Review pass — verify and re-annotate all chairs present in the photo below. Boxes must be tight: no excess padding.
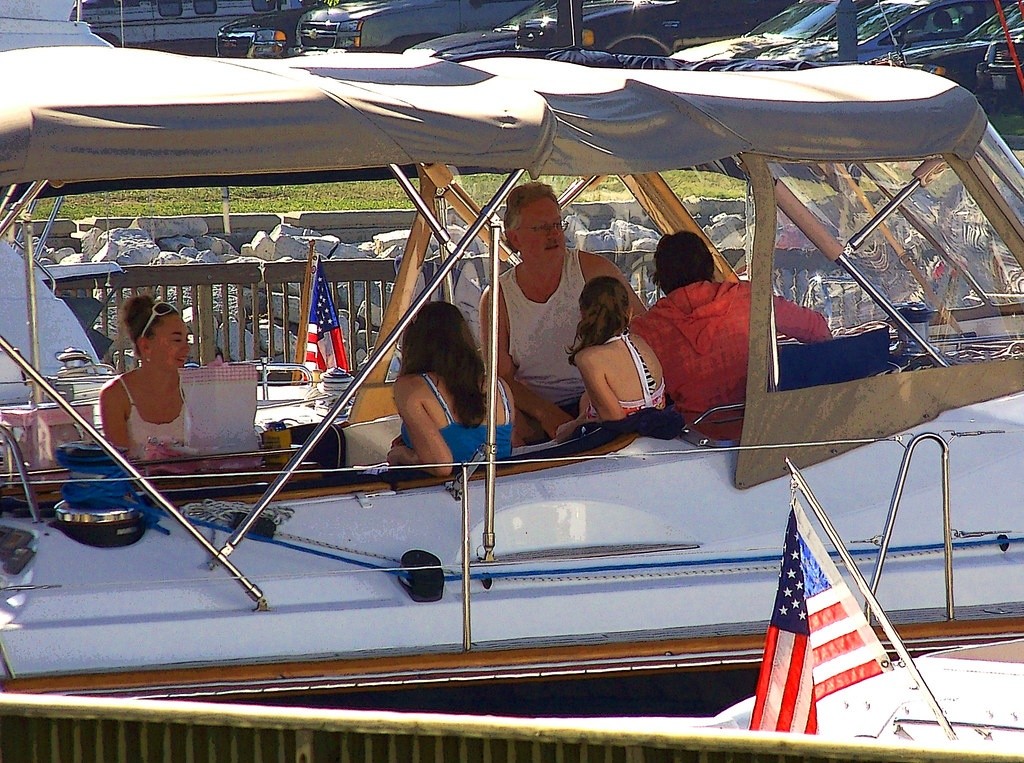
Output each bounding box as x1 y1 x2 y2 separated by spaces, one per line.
772 326 892 394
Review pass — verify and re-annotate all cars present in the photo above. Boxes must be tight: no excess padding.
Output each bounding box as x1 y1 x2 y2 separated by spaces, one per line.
664 0 1024 117
400 0 808 66
215 4 324 57
296 0 550 52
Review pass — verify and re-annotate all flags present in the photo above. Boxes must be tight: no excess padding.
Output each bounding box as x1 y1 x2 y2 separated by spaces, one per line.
302 252 351 380
749 497 892 734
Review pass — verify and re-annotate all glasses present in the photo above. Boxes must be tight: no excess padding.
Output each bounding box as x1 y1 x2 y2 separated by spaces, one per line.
514 220 569 233
141 302 180 338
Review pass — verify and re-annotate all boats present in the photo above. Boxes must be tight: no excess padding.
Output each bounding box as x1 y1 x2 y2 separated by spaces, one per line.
1 40 1024 753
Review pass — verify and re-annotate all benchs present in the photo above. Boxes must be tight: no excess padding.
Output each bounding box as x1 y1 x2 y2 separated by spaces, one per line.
1 406 684 515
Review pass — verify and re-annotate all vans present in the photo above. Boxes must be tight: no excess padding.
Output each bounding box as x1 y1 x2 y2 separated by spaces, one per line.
64 1 327 54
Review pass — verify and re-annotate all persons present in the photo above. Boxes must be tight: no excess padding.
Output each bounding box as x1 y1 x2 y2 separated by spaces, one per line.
632 232 834 441
387 302 516 479
557 275 667 440
100 296 190 450
480 182 645 447
